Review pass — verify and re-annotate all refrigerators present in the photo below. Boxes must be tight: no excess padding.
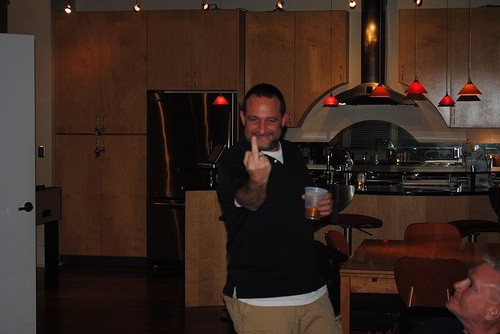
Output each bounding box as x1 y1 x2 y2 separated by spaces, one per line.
146 88 236 295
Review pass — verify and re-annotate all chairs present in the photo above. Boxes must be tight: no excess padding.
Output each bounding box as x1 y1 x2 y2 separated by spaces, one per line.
323 221 472 334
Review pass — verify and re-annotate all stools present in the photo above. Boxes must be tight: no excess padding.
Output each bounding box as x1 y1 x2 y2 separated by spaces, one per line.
329 213 383 247
449 219 500 242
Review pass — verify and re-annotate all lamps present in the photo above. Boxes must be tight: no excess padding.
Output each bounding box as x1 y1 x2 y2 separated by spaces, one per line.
213 92 229 105
369 0 392 98
202 2 217 9
438 0 456 106
63 3 72 14
275 1 284 12
133 2 141 11
320 0 340 107
402 5 428 95
457 0 483 96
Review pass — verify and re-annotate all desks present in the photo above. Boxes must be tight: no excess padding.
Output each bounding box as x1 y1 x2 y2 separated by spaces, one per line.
339 238 500 334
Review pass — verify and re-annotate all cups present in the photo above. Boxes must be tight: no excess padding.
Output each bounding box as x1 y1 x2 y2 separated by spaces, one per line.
357 174 365 191
304 187 329 220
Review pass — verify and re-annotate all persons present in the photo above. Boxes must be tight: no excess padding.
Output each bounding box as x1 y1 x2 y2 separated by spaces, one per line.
408 255 500 334
215 83 340 334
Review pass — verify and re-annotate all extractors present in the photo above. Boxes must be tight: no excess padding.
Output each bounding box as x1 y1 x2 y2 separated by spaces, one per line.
322 0 419 108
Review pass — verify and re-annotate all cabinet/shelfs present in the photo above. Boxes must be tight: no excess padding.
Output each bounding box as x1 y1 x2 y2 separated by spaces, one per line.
50 9 499 310
398 8 500 127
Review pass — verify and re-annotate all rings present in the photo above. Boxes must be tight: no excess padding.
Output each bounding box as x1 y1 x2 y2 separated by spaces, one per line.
259 155 264 157
